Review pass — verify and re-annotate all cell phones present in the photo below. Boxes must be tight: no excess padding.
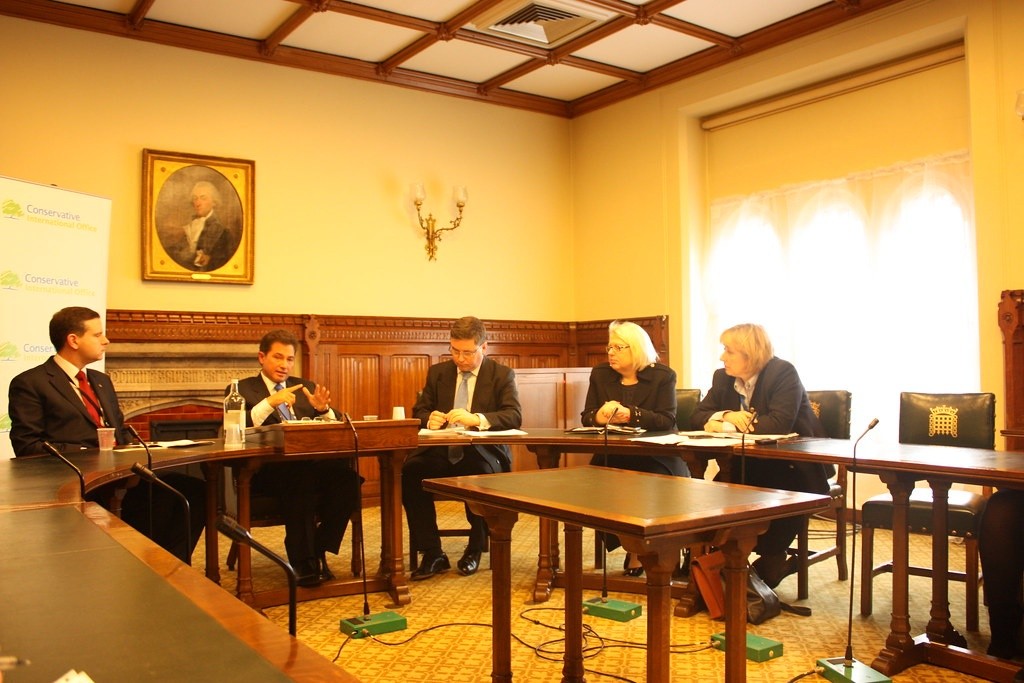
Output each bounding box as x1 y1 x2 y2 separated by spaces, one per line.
756 439 776 444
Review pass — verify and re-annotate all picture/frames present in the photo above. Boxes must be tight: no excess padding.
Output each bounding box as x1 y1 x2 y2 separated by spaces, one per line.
141 148 255 285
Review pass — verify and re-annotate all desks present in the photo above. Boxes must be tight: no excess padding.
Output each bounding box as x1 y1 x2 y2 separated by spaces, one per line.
420 465 831 683
0 418 1024 683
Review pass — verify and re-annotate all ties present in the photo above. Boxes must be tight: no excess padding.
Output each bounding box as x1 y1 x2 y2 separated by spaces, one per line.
275 383 294 422
76 370 101 426
448 371 473 464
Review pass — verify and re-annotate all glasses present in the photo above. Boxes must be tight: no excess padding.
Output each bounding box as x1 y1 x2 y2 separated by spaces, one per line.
605 344 630 354
448 344 485 359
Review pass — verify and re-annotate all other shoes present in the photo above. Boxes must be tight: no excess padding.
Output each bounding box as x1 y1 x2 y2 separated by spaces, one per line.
299 573 321 588
316 550 336 578
627 551 645 578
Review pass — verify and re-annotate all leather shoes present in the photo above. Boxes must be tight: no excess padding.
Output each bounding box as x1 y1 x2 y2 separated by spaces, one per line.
410 549 450 580
457 546 482 576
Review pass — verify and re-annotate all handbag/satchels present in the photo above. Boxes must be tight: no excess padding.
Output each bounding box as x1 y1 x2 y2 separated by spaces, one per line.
693 544 725 619
744 564 813 625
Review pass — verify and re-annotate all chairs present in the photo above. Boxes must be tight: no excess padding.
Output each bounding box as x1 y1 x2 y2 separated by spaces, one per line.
214 384 996 633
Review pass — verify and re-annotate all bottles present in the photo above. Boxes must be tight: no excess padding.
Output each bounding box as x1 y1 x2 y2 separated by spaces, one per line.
224 379 246 442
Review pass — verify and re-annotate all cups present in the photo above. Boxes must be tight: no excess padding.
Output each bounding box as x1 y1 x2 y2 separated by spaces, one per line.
363 415 378 421
393 406 405 420
223 424 242 447
97 428 116 450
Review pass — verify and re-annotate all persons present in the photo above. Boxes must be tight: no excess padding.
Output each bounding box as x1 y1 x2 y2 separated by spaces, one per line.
689 323 837 576
9 306 207 559
402 316 523 580
581 322 691 576
225 330 358 586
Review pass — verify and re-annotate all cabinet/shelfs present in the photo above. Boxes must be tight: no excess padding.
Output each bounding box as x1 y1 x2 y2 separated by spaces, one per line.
512 367 599 474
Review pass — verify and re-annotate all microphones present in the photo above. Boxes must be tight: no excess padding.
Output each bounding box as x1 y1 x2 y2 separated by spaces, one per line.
43 425 298 636
602 408 618 603
344 411 369 616
741 412 757 484
845 418 879 665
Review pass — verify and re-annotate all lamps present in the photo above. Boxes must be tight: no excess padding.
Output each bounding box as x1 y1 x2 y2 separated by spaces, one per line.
411 183 468 260
